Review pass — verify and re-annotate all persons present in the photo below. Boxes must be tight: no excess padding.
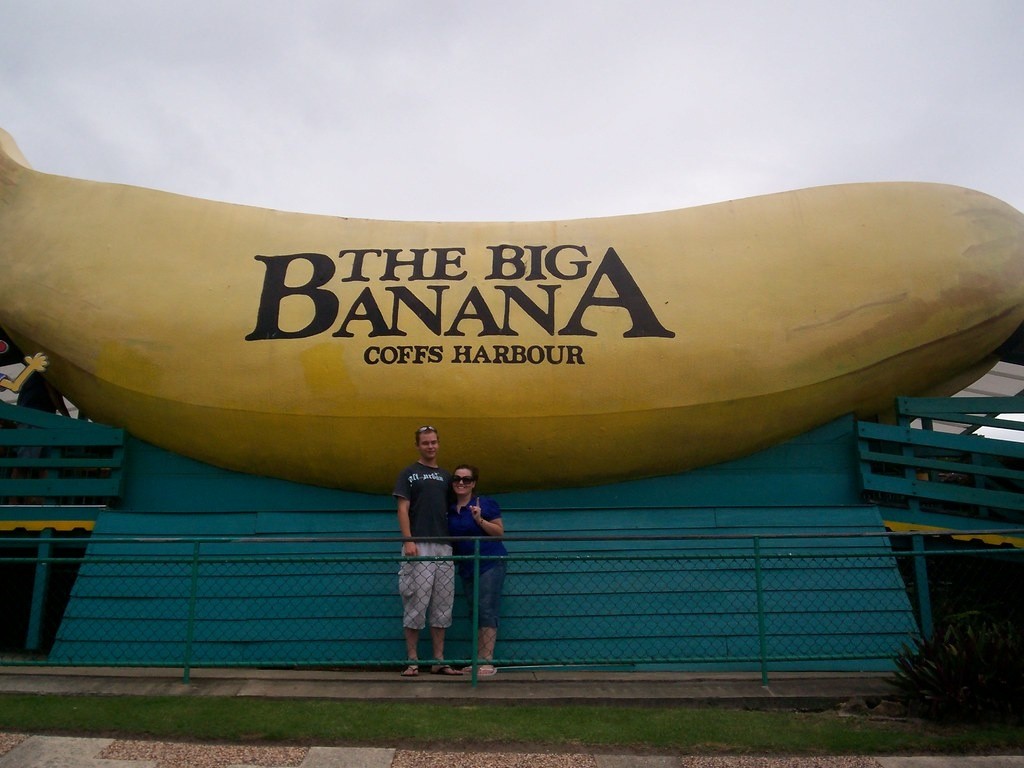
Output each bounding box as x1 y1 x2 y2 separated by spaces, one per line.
9 368 72 504
448 463 507 677
392 424 461 676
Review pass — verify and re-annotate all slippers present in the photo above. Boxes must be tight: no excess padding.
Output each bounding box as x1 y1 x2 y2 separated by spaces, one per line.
404 667 419 676
463 666 472 674
477 668 497 676
431 666 463 675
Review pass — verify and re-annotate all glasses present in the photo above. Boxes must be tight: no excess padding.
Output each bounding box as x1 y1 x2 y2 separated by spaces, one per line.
451 476 474 484
418 426 437 432
